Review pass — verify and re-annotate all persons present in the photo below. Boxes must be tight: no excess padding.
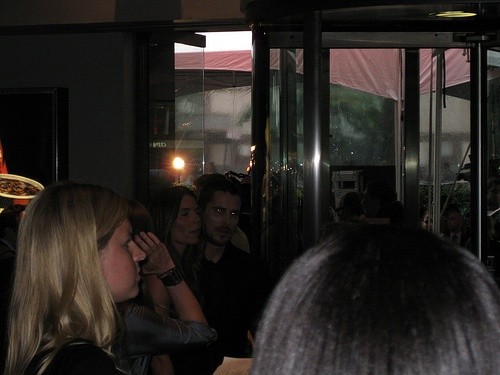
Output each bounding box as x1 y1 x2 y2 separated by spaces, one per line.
0 172 251 375
253 223 500 375
442 172 500 250
